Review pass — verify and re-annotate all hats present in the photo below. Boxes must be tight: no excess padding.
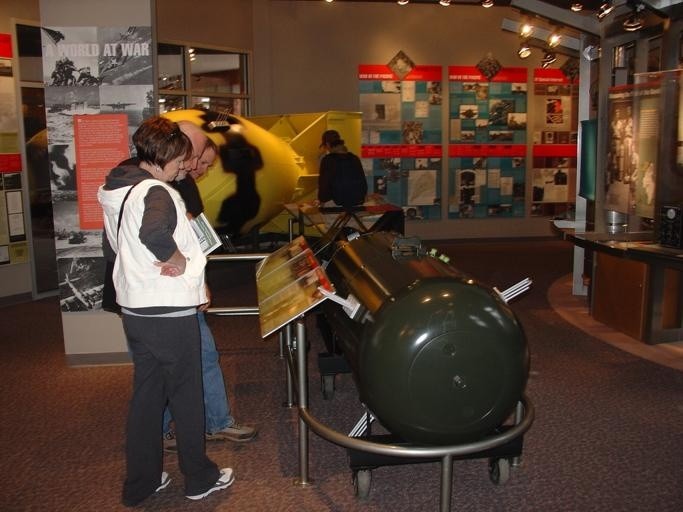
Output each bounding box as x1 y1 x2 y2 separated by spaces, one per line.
318 130 340 149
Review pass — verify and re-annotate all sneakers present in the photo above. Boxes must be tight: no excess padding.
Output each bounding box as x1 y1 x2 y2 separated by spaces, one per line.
121 472 172 507
185 467 236 501
162 430 178 454
206 420 257 443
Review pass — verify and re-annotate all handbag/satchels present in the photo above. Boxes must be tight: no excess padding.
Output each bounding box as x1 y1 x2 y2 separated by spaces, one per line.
101 248 121 315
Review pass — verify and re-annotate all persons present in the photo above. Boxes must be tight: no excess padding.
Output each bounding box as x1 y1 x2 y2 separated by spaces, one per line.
621 105 634 184
189 137 219 182
49 56 98 87
609 108 622 184
163 120 258 455
95 116 236 502
623 145 640 187
315 128 368 208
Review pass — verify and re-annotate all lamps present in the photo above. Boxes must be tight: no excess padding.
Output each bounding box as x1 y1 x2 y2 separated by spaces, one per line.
396 0 645 66
